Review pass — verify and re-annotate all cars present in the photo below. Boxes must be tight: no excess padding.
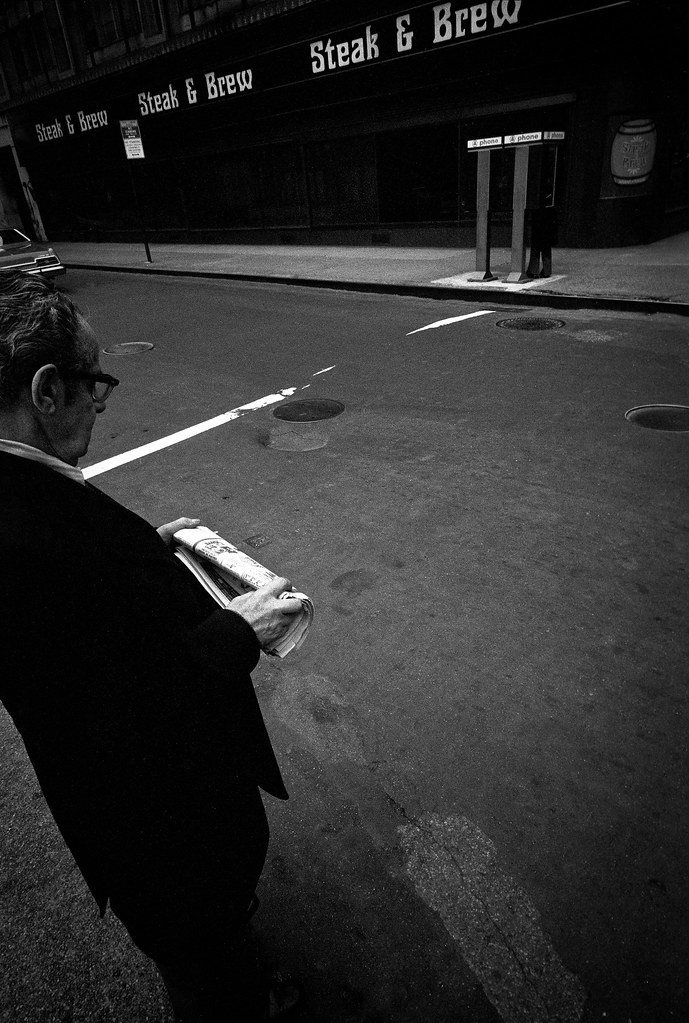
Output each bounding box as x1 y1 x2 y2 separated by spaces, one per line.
0 227 67 287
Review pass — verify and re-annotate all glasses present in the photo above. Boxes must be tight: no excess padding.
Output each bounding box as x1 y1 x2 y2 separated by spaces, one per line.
58 367 120 402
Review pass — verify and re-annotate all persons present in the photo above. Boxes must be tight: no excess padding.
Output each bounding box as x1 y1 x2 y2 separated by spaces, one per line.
0 266 311 1021
